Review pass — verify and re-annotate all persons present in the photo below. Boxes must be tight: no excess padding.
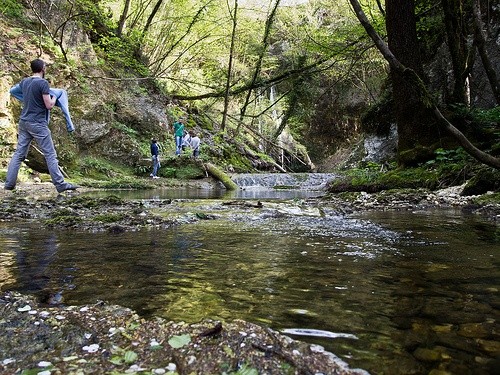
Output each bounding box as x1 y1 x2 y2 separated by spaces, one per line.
191 133 200 157
182 130 193 149
150 139 161 179
172 117 184 156
4 59 78 193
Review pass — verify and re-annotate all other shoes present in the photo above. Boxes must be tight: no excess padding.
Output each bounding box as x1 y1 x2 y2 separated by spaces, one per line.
150 173 160 178
4 187 14 191
56 183 81 193
